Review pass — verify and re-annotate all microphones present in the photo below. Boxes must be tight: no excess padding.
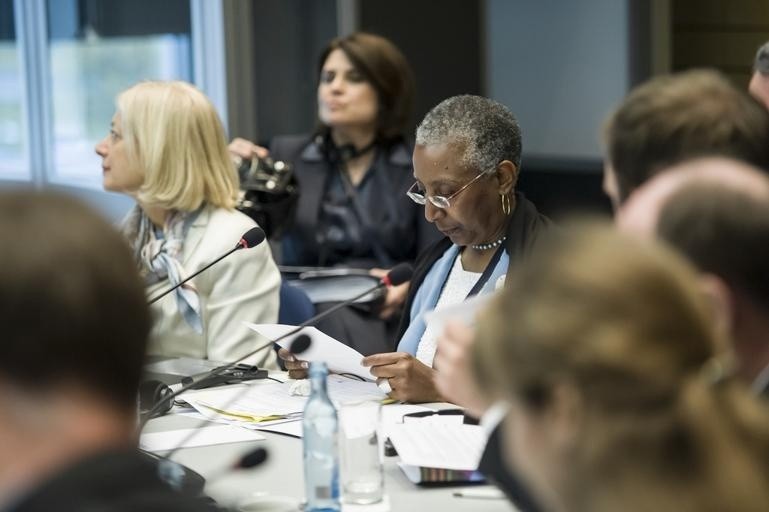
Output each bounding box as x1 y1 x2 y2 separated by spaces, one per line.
131 262 413 450
165 334 311 460
208 446 266 485
145 226 266 309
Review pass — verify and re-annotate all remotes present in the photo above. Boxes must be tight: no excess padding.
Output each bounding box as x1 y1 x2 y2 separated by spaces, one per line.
182 364 268 388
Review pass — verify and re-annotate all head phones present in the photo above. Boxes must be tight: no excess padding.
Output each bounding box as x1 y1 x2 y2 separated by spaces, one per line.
140 380 175 423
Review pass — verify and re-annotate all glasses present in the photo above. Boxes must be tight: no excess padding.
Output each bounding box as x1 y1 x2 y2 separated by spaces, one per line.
407 169 487 209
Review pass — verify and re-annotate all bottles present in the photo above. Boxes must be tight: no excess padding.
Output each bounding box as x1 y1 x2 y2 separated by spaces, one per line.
302 362 340 512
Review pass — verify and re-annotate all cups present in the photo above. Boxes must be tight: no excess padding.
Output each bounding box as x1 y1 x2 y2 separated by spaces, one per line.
341 403 384 505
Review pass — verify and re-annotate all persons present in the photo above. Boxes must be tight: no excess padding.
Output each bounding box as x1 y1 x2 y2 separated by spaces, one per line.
431 47 767 511
228 35 442 269
360 96 545 405
2 180 229 511
96 80 281 356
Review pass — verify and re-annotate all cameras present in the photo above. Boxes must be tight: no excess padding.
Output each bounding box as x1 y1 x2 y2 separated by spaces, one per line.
234 153 299 239
